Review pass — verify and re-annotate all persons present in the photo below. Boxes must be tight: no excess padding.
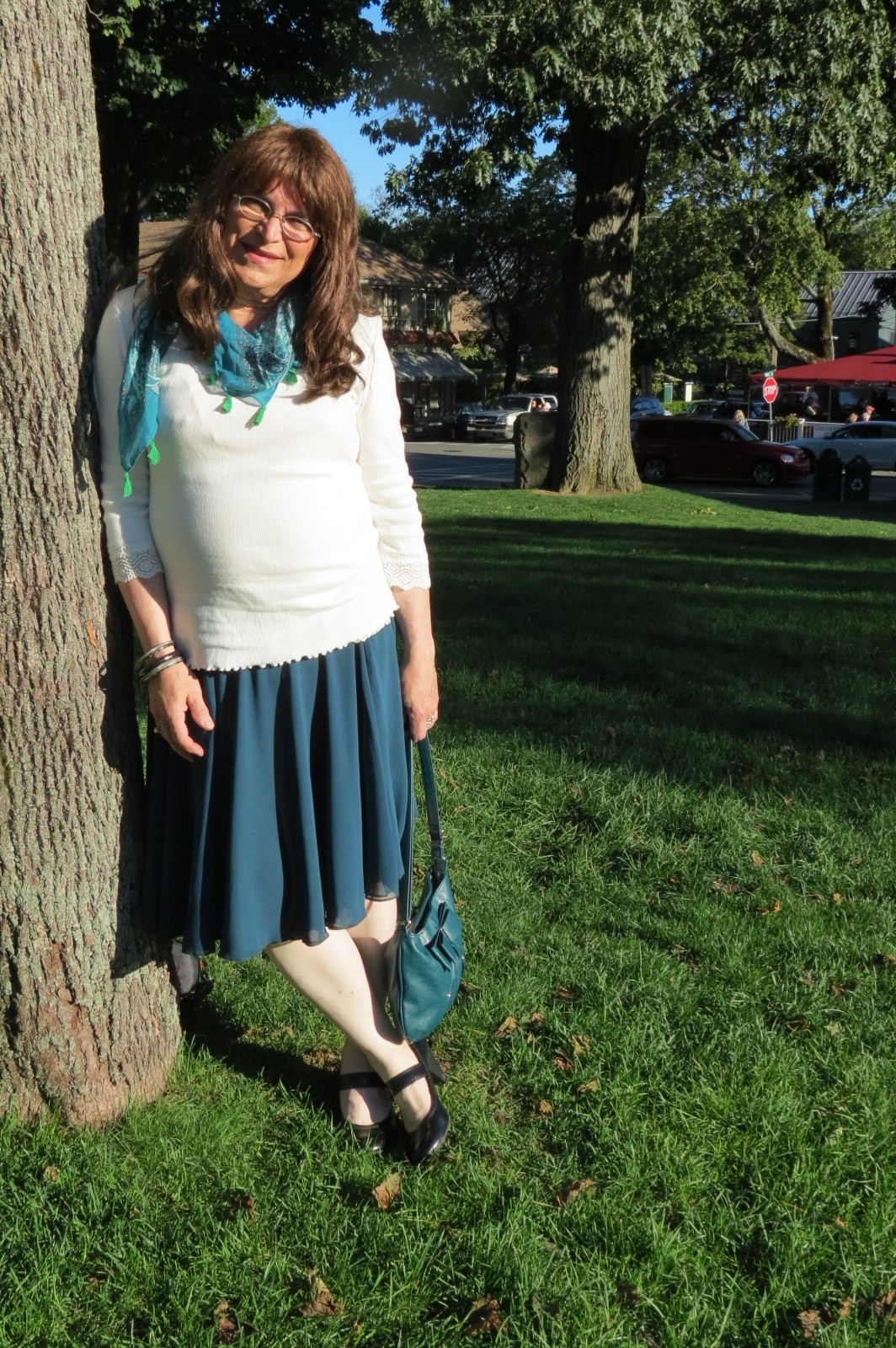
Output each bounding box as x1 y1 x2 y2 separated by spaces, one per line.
530 400 552 413
85 126 454 1164
735 390 890 431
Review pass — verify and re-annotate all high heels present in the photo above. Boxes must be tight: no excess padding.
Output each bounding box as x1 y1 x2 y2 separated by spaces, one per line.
385 1039 449 1166
336 1070 393 1153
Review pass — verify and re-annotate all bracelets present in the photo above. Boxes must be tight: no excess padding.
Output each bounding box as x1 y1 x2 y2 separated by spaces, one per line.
131 641 184 684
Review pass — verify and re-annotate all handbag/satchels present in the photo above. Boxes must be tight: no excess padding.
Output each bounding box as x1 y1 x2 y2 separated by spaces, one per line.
384 729 464 1046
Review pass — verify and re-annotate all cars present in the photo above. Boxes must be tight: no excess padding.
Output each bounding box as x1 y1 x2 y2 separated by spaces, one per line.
629 414 809 491
629 394 665 420
683 398 769 420
781 420 896 475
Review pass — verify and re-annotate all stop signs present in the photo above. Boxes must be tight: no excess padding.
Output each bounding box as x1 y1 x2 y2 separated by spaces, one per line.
762 377 779 403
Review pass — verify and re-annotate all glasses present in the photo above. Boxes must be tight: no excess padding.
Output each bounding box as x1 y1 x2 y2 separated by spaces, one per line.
229 187 322 242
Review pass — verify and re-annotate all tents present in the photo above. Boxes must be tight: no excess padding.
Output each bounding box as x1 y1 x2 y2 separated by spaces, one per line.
753 347 896 420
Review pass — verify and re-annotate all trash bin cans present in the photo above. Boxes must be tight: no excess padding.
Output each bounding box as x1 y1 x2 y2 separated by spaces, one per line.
845 463 871 502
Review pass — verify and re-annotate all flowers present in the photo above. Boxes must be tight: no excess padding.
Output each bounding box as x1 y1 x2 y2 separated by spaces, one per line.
774 412 800 430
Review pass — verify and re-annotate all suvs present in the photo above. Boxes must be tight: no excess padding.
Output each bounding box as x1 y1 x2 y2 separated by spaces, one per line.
455 392 559 444
